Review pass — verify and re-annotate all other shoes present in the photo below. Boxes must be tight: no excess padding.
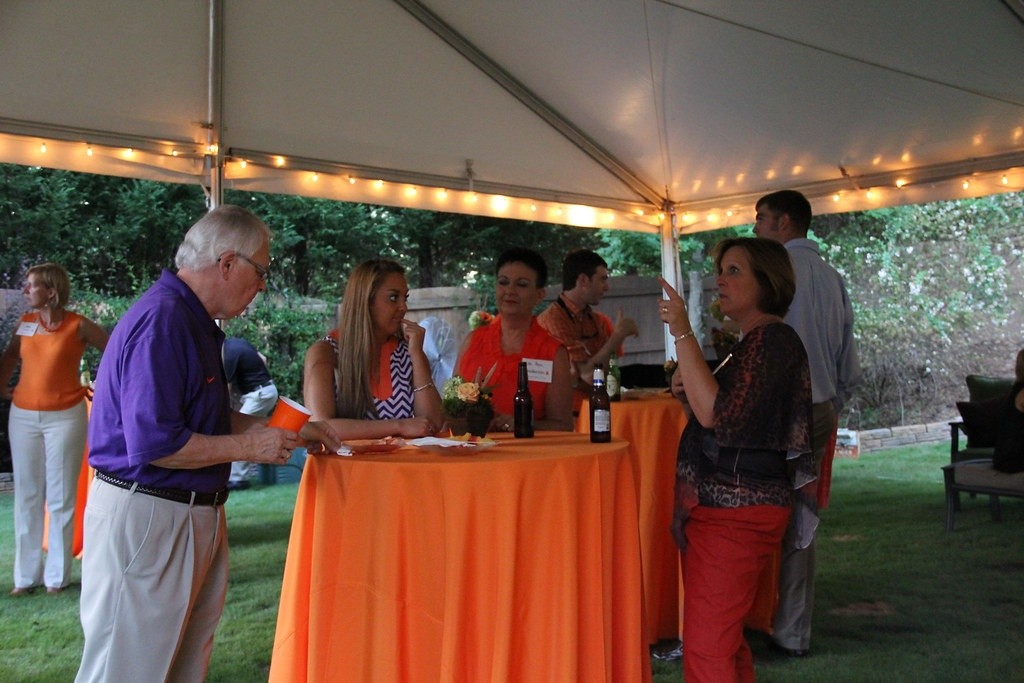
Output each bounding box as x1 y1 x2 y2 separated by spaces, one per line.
10 586 39 596
780 647 808 659
46 586 63 595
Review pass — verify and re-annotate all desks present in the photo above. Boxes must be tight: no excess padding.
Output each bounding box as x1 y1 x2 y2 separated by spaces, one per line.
576 382 690 645
268 428 651 683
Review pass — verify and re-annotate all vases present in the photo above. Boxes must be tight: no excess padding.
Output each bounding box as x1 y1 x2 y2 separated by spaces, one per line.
441 398 493 437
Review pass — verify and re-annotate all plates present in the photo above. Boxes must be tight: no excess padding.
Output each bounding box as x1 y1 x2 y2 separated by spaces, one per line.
409 437 503 456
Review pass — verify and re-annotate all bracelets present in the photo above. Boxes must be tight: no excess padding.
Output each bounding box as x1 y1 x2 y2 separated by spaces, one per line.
674 332 694 344
414 381 435 392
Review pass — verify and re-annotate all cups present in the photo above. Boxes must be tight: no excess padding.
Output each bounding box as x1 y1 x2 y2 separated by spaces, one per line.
266 395 313 432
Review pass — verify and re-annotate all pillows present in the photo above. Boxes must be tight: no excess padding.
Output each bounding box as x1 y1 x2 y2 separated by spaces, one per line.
955 390 1019 448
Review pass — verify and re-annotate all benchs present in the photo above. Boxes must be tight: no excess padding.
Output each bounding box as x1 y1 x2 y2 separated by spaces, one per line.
940 457 1024 534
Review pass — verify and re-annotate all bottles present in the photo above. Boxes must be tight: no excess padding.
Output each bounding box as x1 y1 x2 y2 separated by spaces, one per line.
589 362 612 443
604 353 621 402
512 362 535 438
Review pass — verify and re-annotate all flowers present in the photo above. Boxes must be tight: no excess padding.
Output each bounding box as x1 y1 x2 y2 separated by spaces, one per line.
467 310 496 333
441 360 500 403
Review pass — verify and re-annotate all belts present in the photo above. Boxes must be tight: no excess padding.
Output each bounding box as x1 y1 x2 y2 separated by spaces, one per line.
95 470 230 508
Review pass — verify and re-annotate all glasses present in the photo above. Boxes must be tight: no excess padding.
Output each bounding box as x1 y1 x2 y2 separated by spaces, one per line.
579 317 599 340
218 249 270 281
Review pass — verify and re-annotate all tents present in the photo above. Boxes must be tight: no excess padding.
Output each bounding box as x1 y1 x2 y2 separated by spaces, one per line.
1 0 1024 362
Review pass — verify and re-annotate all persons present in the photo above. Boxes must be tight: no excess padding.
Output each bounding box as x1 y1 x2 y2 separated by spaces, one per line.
452 246 575 432
222 338 277 489
992 349 1024 473
0 264 108 596
653 190 856 660
536 250 640 422
304 259 444 439
657 238 817 683
75 206 344 683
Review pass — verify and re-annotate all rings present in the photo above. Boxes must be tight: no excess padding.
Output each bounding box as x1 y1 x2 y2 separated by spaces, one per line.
663 307 668 313
429 428 433 432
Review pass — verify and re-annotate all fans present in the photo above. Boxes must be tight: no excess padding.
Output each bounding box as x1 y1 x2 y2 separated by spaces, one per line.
418 313 457 390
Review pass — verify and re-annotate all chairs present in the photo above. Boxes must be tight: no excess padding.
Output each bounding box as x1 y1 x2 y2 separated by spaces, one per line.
946 374 1024 507
